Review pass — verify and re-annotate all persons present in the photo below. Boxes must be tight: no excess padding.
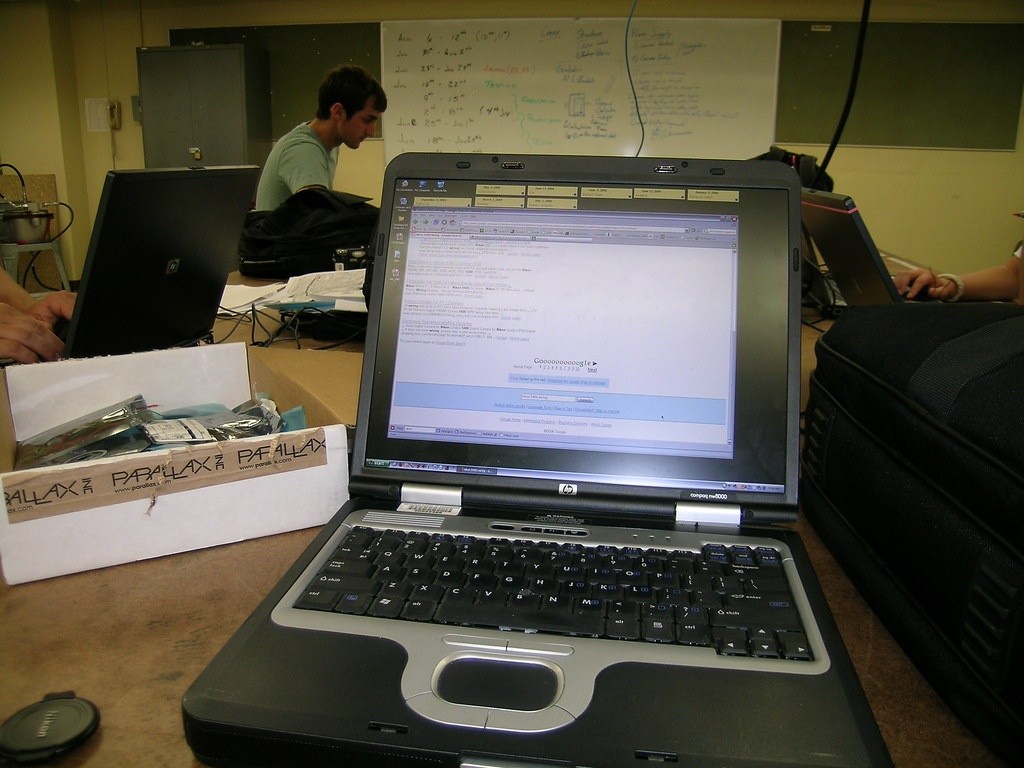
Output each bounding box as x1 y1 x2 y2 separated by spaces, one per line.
893 245 1024 302
0 266 78 364
256 64 388 211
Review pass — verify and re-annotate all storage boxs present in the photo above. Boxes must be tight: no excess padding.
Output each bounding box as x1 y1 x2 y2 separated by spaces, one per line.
0 344 351 585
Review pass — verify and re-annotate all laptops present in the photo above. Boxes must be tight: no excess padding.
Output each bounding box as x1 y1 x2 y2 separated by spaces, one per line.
179 150 893 768
800 224 848 320
798 186 947 306
60 164 261 361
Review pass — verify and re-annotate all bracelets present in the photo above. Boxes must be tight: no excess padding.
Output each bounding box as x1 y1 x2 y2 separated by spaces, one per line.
938 273 964 302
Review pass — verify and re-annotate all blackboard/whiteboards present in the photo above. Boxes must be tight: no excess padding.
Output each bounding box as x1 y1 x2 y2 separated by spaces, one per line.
168 19 383 141
380 18 783 161
773 18 1024 152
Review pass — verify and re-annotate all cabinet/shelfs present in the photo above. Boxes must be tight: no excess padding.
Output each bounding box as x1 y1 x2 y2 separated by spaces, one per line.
134 44 272 167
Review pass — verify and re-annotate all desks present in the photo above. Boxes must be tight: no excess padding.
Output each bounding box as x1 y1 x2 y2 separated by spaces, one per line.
0 272 1024 768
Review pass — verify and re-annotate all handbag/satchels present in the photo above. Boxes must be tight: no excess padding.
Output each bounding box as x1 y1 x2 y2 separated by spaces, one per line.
240 188 384 280
